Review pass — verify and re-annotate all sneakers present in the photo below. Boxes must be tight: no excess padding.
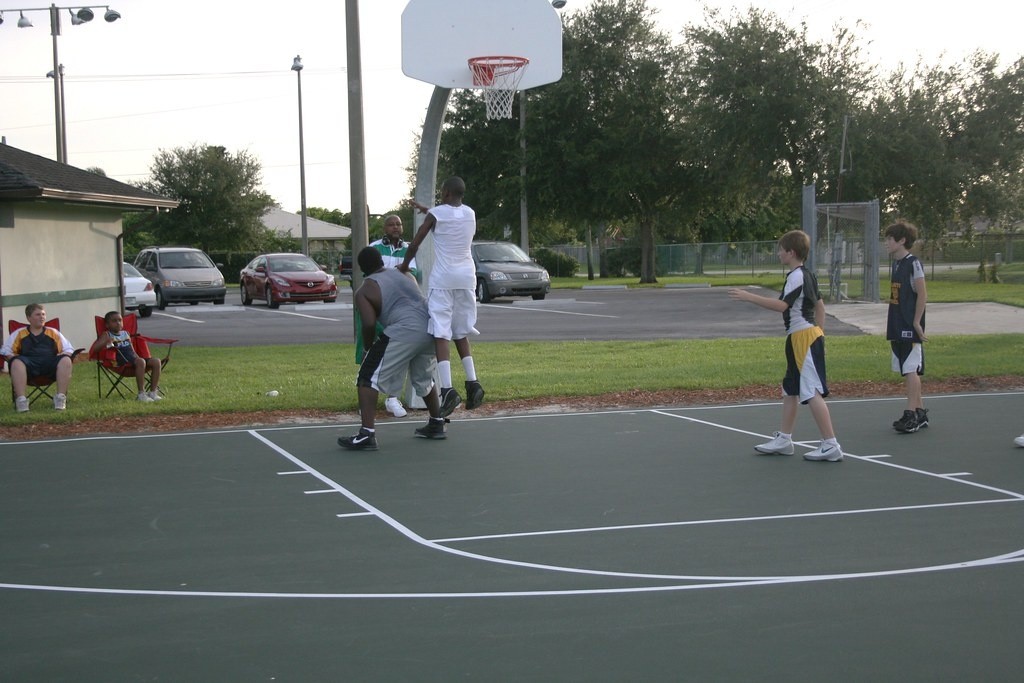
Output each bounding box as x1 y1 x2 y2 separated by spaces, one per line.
895 409 920 433
16 398 29 412
892 407 930 428
465 381 485 409
136 393 154 402
384 399 407 417
414 417 450 439
439 389 462 417
53 392 67 410
337 427 379 451
148 390 161 400
803 439 844 461
754 431 795 455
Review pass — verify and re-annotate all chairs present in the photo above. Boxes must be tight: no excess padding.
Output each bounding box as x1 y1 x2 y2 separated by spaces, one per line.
94 314 179 402
8 318 82 410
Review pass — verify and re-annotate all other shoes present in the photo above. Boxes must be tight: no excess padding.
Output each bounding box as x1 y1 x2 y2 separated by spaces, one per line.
1015 434 1024 446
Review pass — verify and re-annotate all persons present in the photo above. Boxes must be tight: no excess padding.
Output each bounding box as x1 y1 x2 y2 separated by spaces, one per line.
726 230 845 462
92 311 163 403
396 176 484 417
335 247 450 450
0 303 76 413
354 215 419 417
883 221 932 434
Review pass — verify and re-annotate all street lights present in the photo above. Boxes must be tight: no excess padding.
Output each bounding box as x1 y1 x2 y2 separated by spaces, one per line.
291 53 310 258
519 0 567 259
0 3 123 165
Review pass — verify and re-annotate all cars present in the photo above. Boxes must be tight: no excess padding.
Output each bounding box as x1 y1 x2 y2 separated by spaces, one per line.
471 241 552 305
241 253 339 308
122 260 158 318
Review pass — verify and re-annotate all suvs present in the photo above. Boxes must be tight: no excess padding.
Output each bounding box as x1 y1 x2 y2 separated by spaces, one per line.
131 245 227 311
